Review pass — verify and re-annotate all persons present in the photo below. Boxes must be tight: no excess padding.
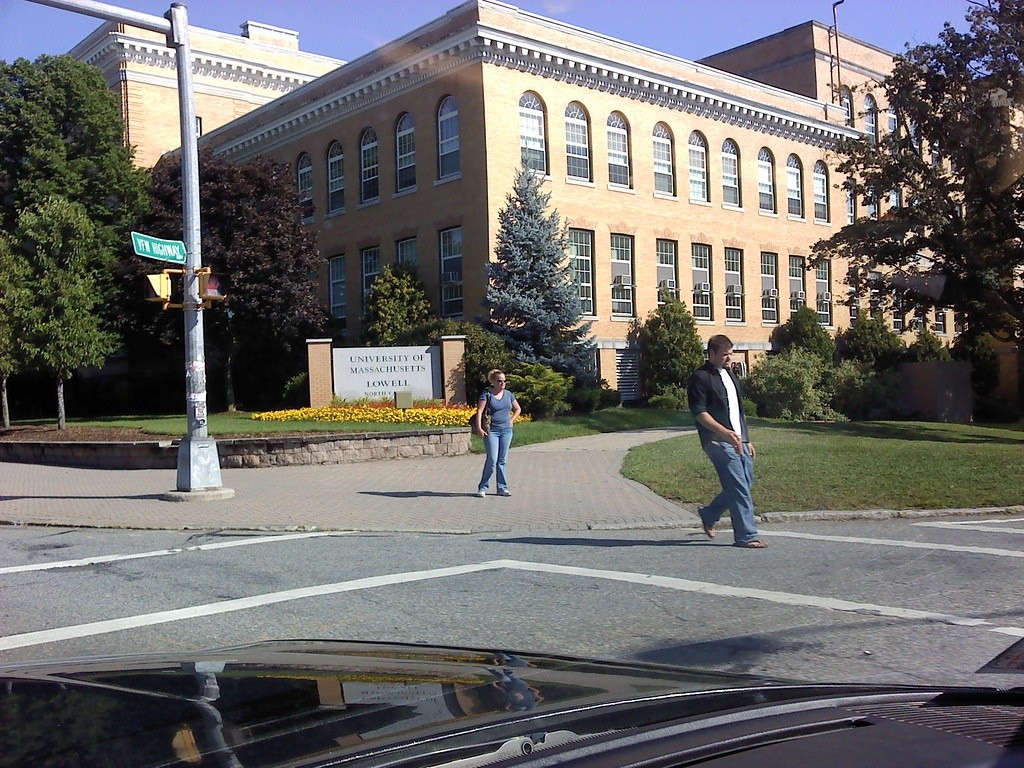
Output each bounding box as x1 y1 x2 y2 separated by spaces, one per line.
687 332 767 548
475 369 522 497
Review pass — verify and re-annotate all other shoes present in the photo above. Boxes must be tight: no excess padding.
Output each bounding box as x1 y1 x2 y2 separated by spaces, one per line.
699 509 716 538
498 489 511 496
732 540 769 548
477 490 486 498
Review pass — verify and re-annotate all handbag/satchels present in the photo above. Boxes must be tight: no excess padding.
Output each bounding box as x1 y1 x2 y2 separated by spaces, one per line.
468 388 491 436
